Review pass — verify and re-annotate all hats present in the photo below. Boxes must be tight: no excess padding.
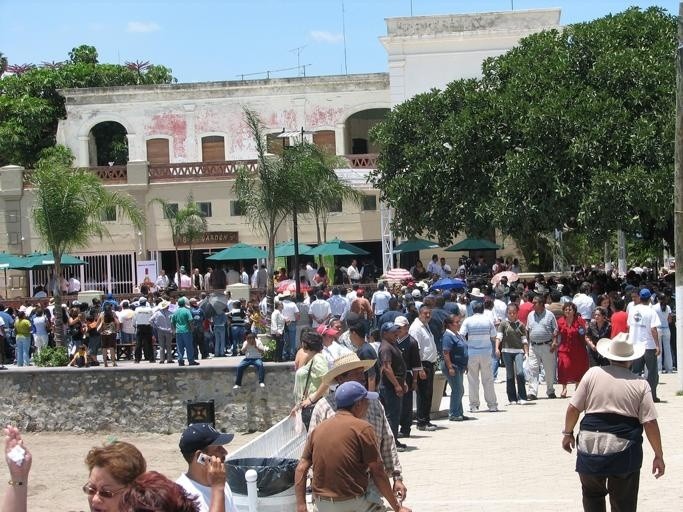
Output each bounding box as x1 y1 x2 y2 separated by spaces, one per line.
72 300 82 305
189 298 196 302
93 298 101 305
157 300 171 310
556 284 565 292
533 286 550 294
408 282 414 286
411 289 421 297
662 287 672 295
595 332 646 362
468 288 486 298
639 288 651 299
277 293 284 298
104 302 112 307
334 381 379 409
347 312 358 319
180 266 185 271
107 294 111 298
139 300 146 304
623 284 635 291
139 297 148 301
580 282 591 294
36 303 42 307
317 324 340 337
380 322 399 338
283 290 291 296
357 290 364 294
394 316 409 327
178 423 234 453
233 300 241 306
324 352 377 384
322 315 337 325
402 294 414 301
178 298 184 305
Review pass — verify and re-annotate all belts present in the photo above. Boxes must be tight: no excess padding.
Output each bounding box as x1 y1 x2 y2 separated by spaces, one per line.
449 361 469 375
528 338 553 345
312 495 366 502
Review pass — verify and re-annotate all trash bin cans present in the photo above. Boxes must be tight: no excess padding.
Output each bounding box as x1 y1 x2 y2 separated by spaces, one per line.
223 458 299 511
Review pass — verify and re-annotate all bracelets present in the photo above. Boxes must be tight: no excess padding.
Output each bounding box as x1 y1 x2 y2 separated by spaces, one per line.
307 396 313 404
562 431 573 436
7 479 27 486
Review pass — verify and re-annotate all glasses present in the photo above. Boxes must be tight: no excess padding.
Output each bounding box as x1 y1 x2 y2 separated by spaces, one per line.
595 314 601 316
320 325 331 335
82 483 129 497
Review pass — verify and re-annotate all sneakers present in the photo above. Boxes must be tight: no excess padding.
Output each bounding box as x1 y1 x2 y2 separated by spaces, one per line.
395 440 408 451
202 356 213 359
189 361 200 366
178 360 185 366
653 398 661 402
150 359 155 362
26 364 33 366
395 431 410 438
167 361 174 363
277 359 287 362
134 360 139 363
417 421 438 431
527 394 538 400
160 360 164 363
448 412 468 421
561 391 568 399
232 385 241 389
260 383 266 387
470 405 479 413
112 363 117 366
518 395 526 405
549 393 556 398
490 405 498 412
511 401 517 404
105 364 108 366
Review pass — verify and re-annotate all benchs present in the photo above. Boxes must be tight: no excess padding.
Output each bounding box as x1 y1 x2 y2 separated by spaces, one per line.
116 343 177 361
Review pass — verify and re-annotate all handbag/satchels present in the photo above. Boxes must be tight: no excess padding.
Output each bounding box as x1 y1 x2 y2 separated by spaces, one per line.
300 358 320 423
285 361 313 431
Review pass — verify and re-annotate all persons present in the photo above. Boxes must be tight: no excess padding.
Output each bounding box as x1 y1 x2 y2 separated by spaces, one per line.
307 355 407 505
459 301 498 413
0 425 32 512
526 296 558 400
323 314 345 342
458 256 675 301
293 328 319 370
245 262 271 333
584 305 612 367
561 332 666 512
348 321 382 392
0 263 245 367
280 290 300 361
175 422 237 512
439 314 470 421
652 292 673 374
293 332 328 433
394 315 423 438
627 289 662 403
296 259 458 319
293 380 410 512
495 302 529 405
271 302 288 362
409 303 438 431
610 297 628 337
233 334 266 389
83 440 147 512
557 302 586 398
120 471 201 511
377 321 407 452
316 326 355 394
271 261 296 294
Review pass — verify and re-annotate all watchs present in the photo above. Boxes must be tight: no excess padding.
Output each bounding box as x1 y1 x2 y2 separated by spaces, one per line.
392 475 403 480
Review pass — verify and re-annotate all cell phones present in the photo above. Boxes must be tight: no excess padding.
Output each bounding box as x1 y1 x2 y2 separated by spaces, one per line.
196 452 213 465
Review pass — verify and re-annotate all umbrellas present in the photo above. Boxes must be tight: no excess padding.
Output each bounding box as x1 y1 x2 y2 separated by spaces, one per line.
32 249 89 270
304 237 371 256
0 251 33 299
272 238 311 257
205 242 269 260
444 237 501 257
394 236 439 252
19 249 47 269
